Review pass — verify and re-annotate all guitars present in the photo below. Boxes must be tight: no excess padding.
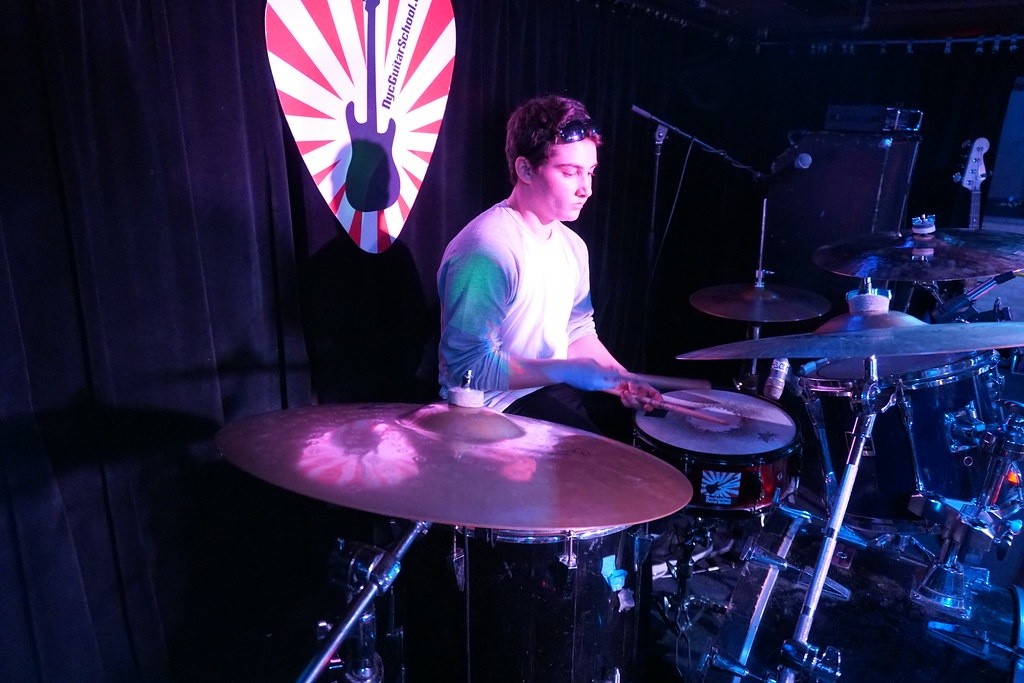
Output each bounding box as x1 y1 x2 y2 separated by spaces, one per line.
951 136 991 231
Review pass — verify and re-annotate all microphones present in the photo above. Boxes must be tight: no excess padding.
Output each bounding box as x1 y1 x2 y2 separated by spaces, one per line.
931 271 1016 324
765 357 789 401
763 154 813 184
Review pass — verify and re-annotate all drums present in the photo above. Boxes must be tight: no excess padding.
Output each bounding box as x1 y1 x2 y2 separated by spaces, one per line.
792 350 1012 539
463 524 640 683
632 386 802 540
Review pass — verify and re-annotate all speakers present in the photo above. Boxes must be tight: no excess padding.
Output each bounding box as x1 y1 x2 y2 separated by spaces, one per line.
758 131 922 335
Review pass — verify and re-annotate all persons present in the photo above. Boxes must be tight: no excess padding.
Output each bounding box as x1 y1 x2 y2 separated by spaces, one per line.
438 96 735 576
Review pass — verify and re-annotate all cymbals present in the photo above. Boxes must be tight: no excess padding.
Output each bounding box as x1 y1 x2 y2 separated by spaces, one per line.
689 276 833 323
674 309 1023 361
807 228 1023 282
214 401 696 534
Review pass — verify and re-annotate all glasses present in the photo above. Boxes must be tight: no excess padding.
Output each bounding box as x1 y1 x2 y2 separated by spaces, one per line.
528 119 599 164
